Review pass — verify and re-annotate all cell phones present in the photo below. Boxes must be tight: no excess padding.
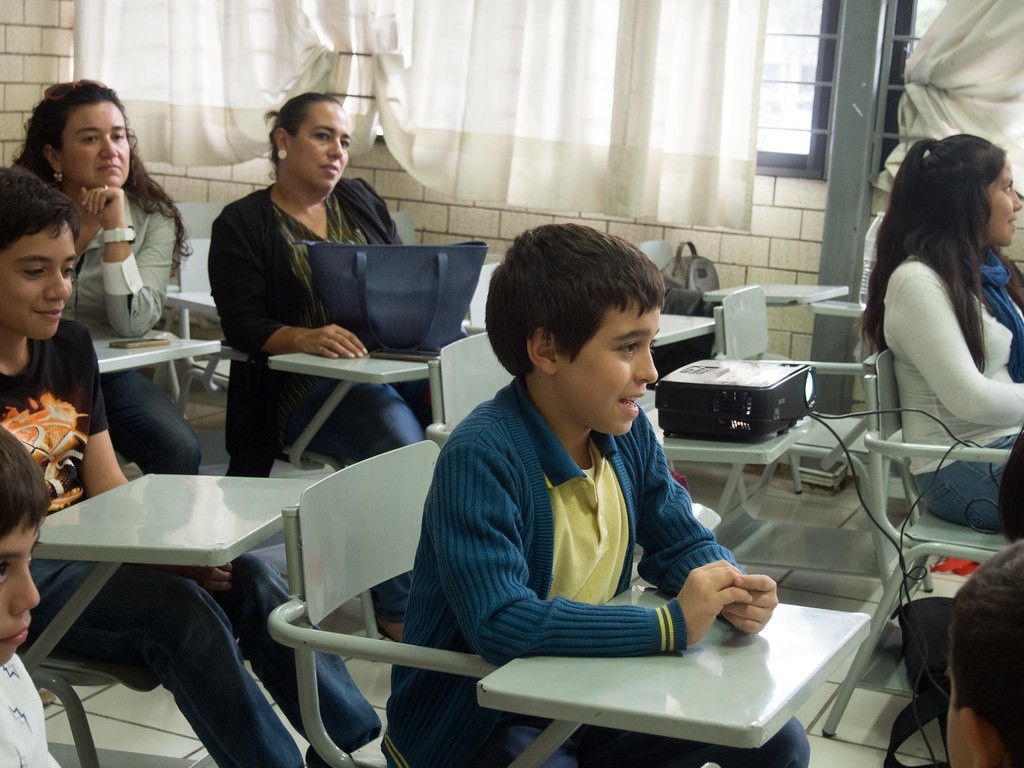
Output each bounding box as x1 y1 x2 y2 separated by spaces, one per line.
109 338 170 348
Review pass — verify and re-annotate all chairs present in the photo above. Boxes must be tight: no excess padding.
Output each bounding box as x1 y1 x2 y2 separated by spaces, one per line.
0 201 1024 768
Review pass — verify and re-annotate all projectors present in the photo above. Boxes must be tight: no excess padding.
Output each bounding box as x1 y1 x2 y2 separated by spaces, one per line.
656 359 816 443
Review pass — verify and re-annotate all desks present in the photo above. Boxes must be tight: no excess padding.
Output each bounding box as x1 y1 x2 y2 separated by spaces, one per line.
164 292 220 391
266 352 430 472
703 282 849 304
645 409 819 517
90 328 222 372
23 473 323 673
651 314 716 347
268 584 871 768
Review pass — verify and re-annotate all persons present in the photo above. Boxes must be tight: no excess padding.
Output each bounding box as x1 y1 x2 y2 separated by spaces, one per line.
378 224 813 768
13 80 200 476
206 91 429 475
860 133 1024 768
1 167 383 767
0 426 64 768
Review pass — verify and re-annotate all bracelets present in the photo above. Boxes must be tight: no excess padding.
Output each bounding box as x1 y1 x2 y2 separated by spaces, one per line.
96 225 138 246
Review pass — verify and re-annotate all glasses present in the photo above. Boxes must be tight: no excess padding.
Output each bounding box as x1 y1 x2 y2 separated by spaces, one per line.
45 79 108 101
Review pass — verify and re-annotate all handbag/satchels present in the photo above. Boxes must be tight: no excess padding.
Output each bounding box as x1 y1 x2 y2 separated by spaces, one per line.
884 596 955 768
657 242 722 316
293 236 489 357
